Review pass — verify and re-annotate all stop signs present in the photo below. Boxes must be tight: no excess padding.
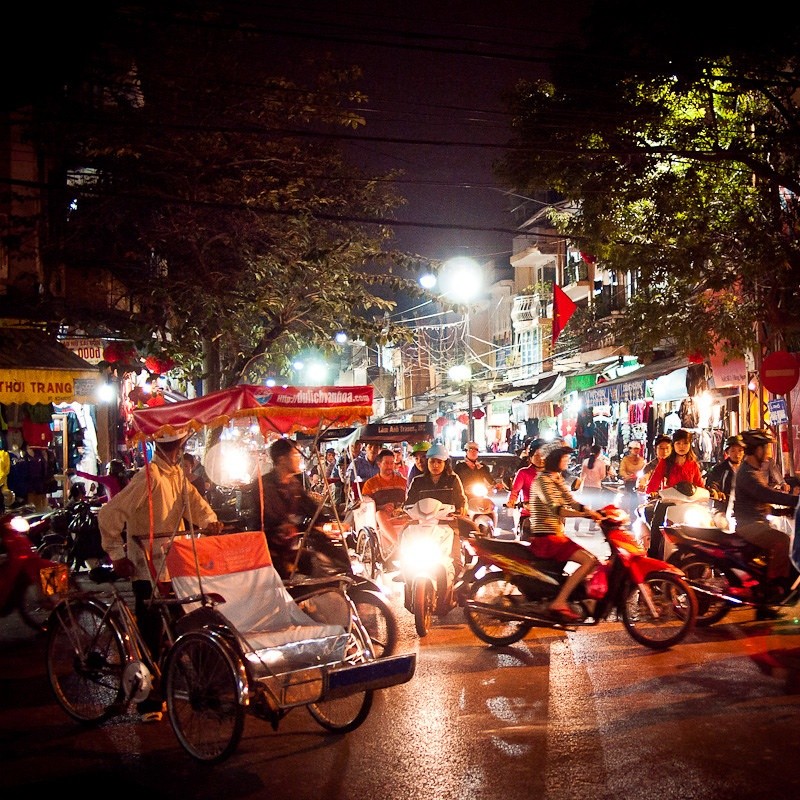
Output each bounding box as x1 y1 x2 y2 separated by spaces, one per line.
759 351 800 396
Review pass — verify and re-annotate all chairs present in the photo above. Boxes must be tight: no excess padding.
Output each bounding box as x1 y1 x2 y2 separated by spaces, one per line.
163 531 348 677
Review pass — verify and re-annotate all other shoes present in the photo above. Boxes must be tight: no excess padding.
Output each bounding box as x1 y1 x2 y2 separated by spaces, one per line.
138 710 163 723
752 607 789 620
761 594 798 607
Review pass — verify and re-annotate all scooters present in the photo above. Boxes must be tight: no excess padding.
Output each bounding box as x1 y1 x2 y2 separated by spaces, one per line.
293 424 800 580
384 497 480 638
1 481 255 638
644 480 723 616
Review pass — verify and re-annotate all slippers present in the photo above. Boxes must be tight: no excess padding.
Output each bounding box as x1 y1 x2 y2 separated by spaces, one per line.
552 607 581 620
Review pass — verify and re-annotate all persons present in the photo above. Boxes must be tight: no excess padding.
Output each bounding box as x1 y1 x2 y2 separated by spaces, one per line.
602 455 621 482
251 439 329 564
705 436 746 530
68 482 86 501
529 440 615 620
620 429 705 557
97 435 224 722
183 452 211 531
66 459 131 504
507 439 550 516
762 456 790 491
733 430 800 597
574 445 605 531
309 440 505 583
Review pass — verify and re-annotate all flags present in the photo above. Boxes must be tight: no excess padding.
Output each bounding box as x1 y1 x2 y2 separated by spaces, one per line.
551 284 578 348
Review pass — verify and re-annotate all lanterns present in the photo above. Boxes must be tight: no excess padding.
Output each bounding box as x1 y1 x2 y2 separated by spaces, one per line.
146 354 175 374
128 387 151 405
436 416 447 426
103 342 138 366
458 414 469 424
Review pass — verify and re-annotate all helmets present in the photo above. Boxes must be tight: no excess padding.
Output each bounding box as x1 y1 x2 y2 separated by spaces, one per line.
464 441 479 449
425 444 449 461
743 430 778 445
409 441 433 456
155 427 188 443
326 448 337 454
723 435 743 452
528 438 550 456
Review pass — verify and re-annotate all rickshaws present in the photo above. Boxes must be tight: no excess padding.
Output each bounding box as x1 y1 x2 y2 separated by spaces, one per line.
40 381 417 769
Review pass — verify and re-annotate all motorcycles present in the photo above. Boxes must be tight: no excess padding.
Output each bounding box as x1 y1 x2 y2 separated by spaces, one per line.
659 502 799 627
457 505 700 649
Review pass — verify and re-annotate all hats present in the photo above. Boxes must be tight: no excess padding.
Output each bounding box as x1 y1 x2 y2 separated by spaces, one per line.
627 441 641 449
655 436 672 445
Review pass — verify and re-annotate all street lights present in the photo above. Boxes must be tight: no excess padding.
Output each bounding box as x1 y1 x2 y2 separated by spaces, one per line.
434 255 489 441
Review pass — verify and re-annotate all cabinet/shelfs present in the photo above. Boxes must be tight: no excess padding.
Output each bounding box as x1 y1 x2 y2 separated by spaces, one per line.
51 414 69 509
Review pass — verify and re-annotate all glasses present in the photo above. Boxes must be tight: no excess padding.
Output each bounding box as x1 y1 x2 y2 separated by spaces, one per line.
468 449 478 451
393 450 401 453
